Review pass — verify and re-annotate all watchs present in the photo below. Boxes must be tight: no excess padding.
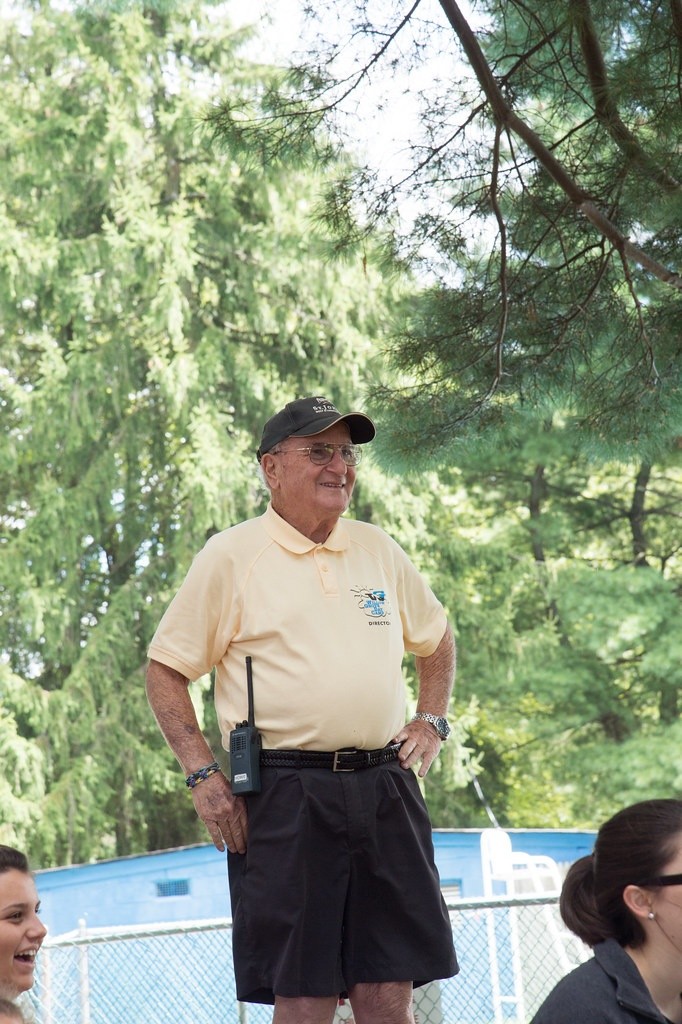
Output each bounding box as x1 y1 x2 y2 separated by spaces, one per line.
412 713 450 741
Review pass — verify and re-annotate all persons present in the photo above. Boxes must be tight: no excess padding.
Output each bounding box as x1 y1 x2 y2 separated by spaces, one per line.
530 799 682 1024
144 395 460 1024
0 845 49 1024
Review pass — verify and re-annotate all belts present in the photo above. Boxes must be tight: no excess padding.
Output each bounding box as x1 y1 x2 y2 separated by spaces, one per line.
261 741 404 774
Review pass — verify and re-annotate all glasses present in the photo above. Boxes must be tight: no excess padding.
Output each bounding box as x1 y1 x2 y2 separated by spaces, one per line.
270 442 363 465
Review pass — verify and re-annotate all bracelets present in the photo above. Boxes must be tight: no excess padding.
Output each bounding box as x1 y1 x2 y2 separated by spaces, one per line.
185 763 220 790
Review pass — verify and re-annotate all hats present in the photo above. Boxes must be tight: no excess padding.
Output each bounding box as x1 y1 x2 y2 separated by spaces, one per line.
255 397 376 464
